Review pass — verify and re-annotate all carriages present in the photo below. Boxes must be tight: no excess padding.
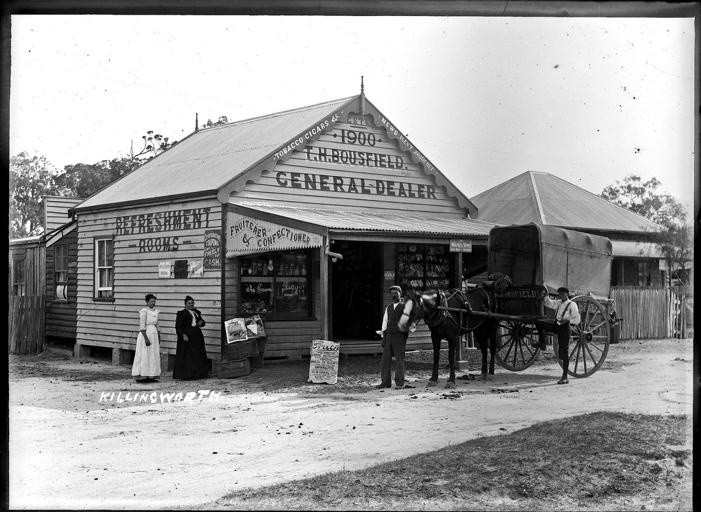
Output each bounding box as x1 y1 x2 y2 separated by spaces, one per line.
396 222 617 389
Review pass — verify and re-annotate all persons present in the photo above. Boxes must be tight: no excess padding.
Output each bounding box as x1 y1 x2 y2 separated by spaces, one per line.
174 295 212 379
376 284 416 390
531 287 581 384
132 293 163 382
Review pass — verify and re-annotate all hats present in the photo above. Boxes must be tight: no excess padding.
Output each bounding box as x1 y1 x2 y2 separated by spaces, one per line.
389 285 402 296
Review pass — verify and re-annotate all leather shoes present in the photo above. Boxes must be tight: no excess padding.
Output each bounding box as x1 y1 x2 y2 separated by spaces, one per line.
557 379 569 384
136 378 158 383
376 385 401 389
531 342 546 351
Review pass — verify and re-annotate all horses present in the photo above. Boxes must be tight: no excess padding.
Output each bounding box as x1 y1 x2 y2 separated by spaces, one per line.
396 283 501 389
